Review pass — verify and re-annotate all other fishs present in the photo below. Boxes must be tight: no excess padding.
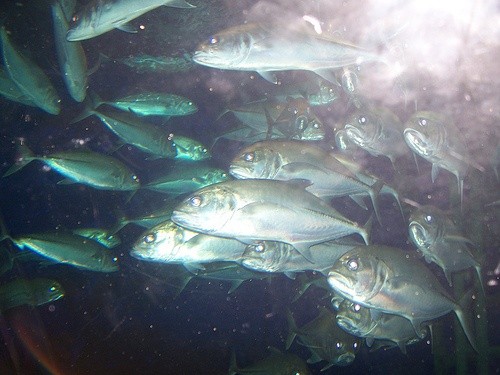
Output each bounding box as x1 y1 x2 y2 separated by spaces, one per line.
0 132 141 191
68 0 196 42
407 205 487 291
133 94 404 298
404 111 488 181
328 245 480 355
98 112 211 157
227 347 307 375
285 308 362 370
1 278 66 309
90 83 199 123
42 0 103 103
0 23 62 115
1 227 123 274
195 22 389 70
104 50 197 73
